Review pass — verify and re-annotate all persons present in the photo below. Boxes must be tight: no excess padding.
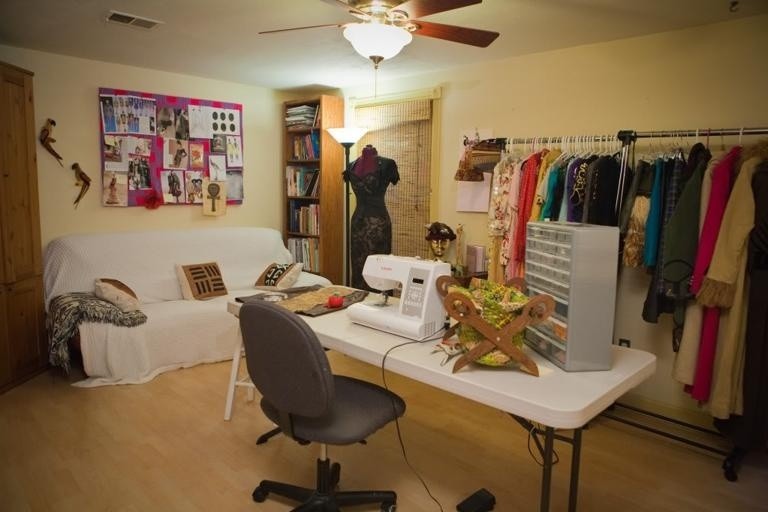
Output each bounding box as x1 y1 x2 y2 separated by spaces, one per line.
425 221 457 262
172 140 189 167
340 145 400 299
105 171 121 204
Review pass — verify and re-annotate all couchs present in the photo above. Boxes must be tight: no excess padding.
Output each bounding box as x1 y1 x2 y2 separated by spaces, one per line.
42 227 332 389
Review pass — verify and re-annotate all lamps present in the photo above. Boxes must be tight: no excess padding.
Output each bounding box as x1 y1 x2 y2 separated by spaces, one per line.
326 127 369 287
343 22 412 70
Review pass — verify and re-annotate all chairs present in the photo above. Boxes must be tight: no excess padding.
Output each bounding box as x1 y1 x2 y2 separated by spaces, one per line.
238 301 405 512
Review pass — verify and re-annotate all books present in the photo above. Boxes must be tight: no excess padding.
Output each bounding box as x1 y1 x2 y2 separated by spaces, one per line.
466 244 486 275
285 105 322 274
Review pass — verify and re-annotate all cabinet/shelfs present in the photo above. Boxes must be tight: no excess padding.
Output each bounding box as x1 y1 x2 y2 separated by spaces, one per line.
283 94 344 284
0 61 51 396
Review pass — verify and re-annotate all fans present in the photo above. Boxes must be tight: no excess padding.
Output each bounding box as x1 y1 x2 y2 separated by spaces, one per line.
259 1 500 48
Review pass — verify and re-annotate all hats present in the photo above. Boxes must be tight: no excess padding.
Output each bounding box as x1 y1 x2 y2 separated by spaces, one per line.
425 223 456 241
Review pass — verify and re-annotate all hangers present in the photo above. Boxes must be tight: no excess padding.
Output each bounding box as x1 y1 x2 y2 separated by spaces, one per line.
506 127 745 162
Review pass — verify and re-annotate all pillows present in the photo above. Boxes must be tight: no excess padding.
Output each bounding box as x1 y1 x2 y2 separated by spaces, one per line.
175 260 229 301
255 262 303 291
94 276 140 313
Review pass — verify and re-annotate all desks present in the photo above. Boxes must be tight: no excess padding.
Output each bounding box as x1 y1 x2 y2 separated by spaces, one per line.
224 285 657 512
